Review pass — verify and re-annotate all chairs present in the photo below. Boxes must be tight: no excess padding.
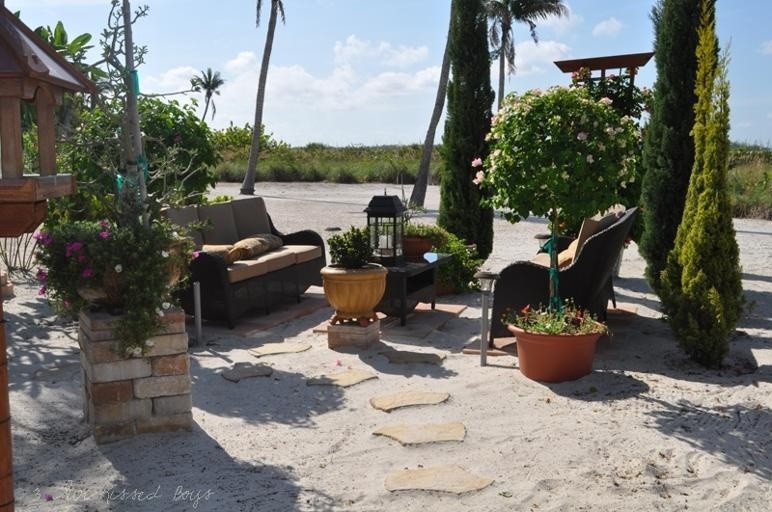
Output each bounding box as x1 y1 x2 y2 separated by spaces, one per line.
489 206 640 350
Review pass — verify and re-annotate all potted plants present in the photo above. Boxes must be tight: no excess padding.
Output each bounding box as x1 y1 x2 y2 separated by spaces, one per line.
404 222 485 296
320 224 388 327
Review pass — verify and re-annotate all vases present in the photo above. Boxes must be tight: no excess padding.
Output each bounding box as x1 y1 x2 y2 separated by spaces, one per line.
508 316 605 383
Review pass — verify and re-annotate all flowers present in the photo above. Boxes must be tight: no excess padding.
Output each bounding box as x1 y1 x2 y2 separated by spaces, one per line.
30 218 201 364
471 84 643 336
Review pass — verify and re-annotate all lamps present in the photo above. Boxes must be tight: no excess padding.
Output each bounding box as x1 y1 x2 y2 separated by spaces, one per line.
363 189 409 268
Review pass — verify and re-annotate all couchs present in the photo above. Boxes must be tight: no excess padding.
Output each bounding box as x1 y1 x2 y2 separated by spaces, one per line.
160 197 327 330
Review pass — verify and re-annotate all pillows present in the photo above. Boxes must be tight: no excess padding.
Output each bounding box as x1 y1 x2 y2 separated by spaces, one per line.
160 197 283 267
558 203 626 271
